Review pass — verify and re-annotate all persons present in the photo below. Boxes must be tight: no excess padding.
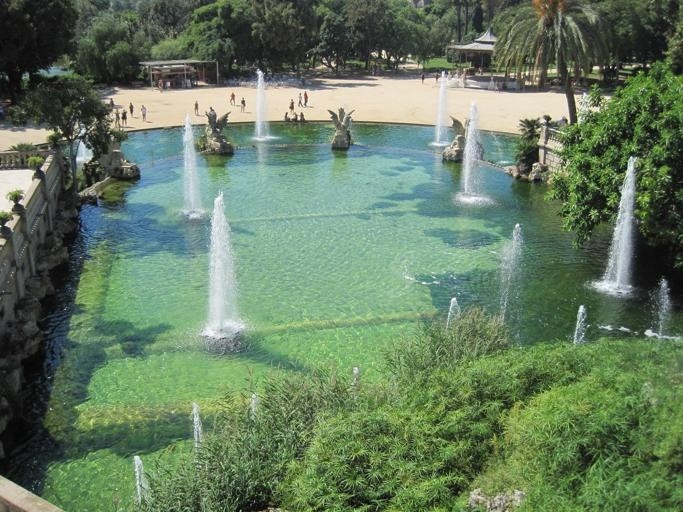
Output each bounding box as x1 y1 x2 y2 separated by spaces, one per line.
240 97 245 113
108 97 127 126
303 91 308 106
158 79 163 95
230 92 235 105
298 92 303 107
496 80 507 94
141 105 147 121
289 99 294 114
129 102 133 117
422 75 424 84
194 100 198 116
